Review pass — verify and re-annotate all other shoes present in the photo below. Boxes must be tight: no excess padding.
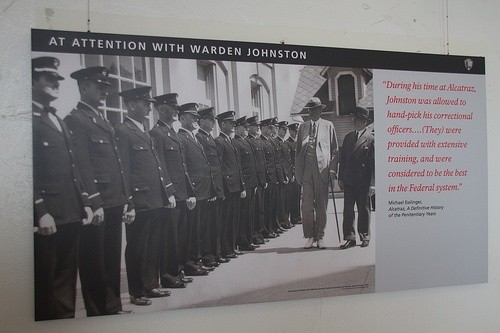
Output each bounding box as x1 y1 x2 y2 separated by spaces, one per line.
304 236 315 248
234 219 303 255
317 240 327 249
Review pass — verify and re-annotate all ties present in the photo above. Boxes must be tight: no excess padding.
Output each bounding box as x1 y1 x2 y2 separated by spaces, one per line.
313 123 317 137
354 132 360 143
98 111 104 121
192 133 196 142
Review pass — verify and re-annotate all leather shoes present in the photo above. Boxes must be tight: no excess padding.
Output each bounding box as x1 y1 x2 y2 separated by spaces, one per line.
226 253 238 258
219 258 230 263
116 310 135 314
146 288 171 298
181 278 193 283
361 240 370 247
130 294 152 305
205 261 220 266
200 265 215 270
161 280 186 288
340 240 356 249
184 268 210 276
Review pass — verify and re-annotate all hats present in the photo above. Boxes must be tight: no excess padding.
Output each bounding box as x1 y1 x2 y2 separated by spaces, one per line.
349 105 371 121
178 103 199 115
152 93 180 107
235 115 299 131
303 97 326 109
118 86 156 103
216 111 236 121
32 56 64 80
197 107 218 119
70 66 112 87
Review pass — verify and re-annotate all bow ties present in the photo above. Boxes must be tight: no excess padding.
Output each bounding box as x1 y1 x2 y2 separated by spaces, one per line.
44 105 58 114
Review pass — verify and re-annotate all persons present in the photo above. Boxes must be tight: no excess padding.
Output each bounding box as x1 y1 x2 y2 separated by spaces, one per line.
178 101 206 278
114 85 176 306
335 107 375 248
214 110 242 258
150 92 193 289
294 97 339 250
231 115 257 252
31 55 94 321
195 106 227 267
65 65 133 316
244 115 303 245
